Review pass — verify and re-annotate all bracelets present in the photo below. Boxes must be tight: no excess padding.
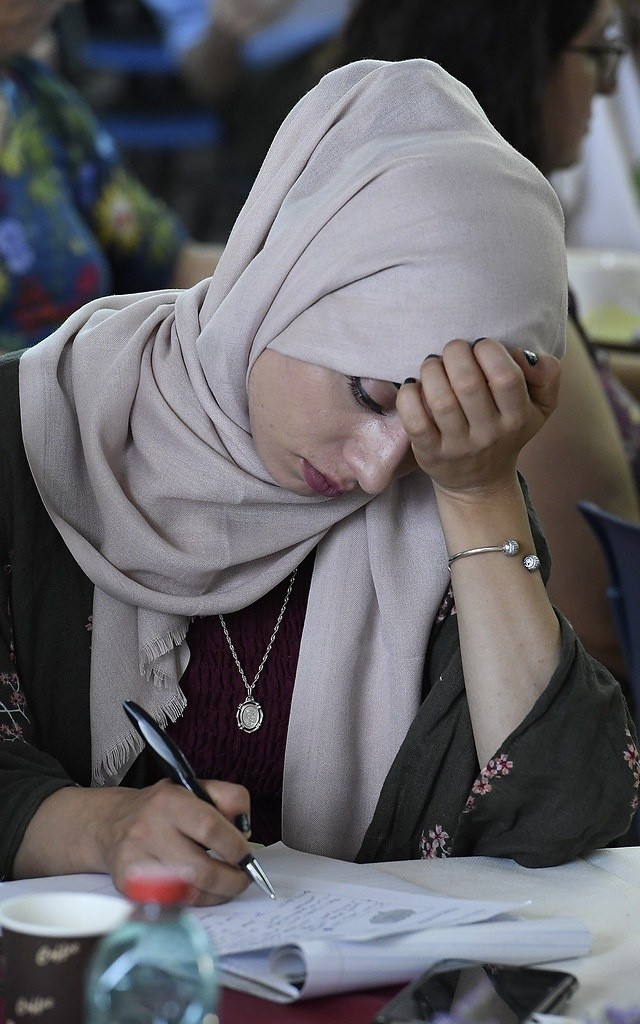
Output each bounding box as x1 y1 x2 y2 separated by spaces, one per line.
450 542 545 573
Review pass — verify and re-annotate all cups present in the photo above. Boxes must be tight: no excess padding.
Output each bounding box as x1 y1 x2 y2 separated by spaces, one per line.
0 893 132 1024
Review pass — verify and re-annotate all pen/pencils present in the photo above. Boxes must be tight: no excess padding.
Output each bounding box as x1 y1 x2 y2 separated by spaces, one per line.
122 698 278 902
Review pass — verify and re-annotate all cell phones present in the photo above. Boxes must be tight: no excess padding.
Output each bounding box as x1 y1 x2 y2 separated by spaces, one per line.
371 959 580 1024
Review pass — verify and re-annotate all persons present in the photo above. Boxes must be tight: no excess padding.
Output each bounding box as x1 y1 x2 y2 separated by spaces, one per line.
0 58 639 907
0 0 640 525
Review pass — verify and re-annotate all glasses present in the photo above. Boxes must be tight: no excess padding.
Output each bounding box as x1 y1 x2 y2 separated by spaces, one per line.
560 19 627 83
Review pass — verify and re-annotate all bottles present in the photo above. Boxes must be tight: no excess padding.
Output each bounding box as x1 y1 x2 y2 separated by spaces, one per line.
86 875 216 1024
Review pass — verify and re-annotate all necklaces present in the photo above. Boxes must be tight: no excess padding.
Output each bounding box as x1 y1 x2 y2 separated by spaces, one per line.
217 569 300 735
